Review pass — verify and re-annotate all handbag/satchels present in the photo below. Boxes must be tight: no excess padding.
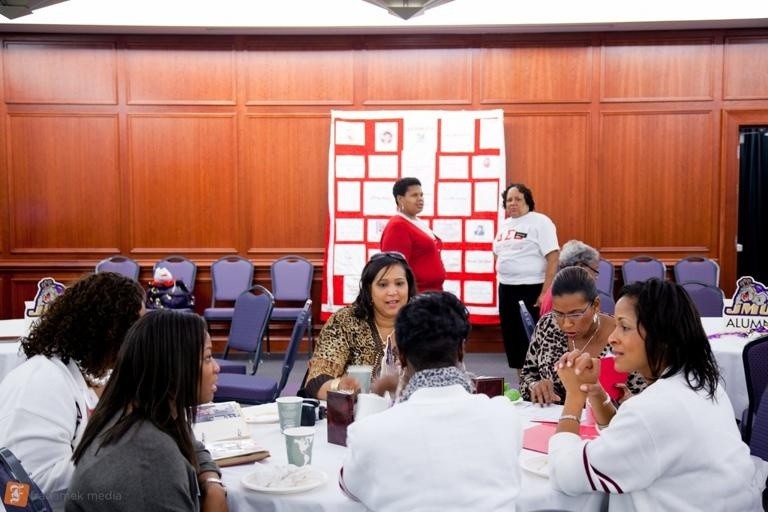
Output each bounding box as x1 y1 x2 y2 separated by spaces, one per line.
146 279 192 309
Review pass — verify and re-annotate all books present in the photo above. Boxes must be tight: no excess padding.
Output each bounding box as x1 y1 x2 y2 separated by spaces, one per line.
185 400 271 468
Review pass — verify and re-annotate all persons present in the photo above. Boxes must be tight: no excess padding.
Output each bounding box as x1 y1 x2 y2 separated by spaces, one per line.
305 249 417 404
337 290 524 512
64 309 230 512
548 278 768 512
491 182 562 384
0 270 147 511
538 239 601 318
379 176 447 292
519 266 653 407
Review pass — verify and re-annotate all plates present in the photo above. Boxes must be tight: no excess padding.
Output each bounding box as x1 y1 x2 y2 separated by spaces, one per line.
242 465 327 494
243 401 278 425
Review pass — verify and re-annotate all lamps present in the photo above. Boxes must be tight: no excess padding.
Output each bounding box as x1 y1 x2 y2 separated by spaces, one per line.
364 1 457 22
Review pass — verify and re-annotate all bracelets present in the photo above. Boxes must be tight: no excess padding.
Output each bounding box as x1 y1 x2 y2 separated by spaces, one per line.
559 414 581 424
586 393 611 407
199 478 228 496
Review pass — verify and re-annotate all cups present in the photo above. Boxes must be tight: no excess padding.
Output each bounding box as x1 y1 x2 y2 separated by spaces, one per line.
276 396 320 466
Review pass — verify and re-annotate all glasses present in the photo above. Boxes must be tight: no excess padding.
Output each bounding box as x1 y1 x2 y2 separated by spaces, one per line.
581 260 600 278
551 301 591 321
371 251 406 262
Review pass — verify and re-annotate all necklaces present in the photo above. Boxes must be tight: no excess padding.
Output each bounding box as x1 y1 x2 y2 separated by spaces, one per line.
571 318 600 354
